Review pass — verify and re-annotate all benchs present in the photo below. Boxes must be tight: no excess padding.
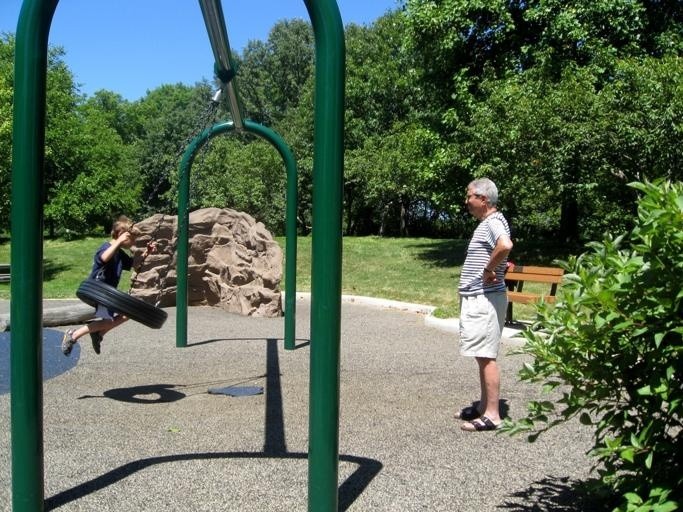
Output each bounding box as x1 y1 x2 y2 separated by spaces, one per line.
498 264 566 326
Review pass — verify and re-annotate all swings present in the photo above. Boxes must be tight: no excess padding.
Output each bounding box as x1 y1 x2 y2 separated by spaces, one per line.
76 60 239 329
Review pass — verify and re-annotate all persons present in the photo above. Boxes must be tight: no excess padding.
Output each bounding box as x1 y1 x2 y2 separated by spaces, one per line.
61 215 158 356
454 176 514 435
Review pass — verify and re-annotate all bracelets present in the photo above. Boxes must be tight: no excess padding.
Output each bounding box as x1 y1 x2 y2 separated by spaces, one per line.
483 266 492 273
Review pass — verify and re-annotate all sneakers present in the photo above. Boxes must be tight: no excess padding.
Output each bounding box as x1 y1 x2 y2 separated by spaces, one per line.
63 328 75 354
89 330 104 354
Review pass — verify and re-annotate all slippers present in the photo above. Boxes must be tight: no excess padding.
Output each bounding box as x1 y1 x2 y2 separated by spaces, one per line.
458 405 479 419
468 416 500 432
